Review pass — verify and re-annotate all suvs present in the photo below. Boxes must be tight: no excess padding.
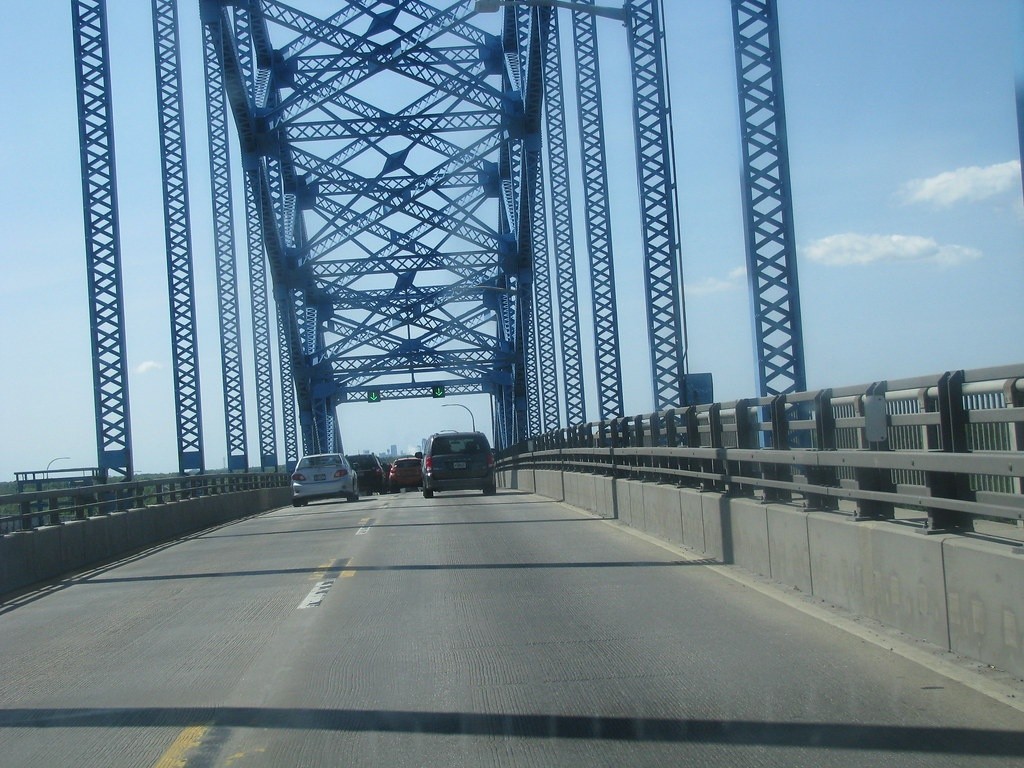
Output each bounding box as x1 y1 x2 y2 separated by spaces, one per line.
345 452 386 496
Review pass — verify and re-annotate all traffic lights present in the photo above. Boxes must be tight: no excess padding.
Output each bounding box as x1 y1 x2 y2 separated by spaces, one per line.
368 390 380 402
433 385 445 398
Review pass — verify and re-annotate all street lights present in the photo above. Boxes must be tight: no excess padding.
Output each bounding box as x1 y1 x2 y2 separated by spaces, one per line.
441 403 475 432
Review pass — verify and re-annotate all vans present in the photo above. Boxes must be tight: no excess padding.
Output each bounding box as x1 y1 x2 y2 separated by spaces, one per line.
414 430 496 498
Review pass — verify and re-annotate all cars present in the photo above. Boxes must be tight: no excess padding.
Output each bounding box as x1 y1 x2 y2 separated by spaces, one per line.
378 456 424 494
290 451 360 507
